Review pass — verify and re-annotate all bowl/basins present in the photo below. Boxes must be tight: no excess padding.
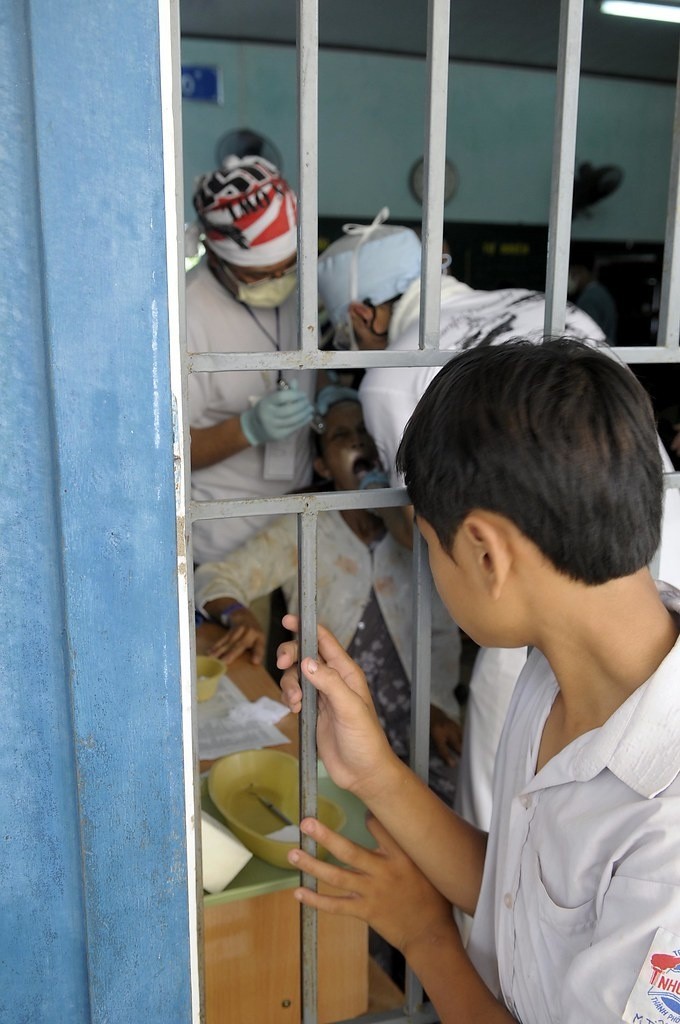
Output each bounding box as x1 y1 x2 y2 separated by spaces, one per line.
196 658 227 700
208 750 347 871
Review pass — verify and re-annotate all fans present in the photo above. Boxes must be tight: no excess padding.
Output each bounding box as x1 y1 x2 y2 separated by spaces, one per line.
572 161 625 223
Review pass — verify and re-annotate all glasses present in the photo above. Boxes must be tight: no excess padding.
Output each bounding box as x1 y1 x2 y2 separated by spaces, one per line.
333 297 371 350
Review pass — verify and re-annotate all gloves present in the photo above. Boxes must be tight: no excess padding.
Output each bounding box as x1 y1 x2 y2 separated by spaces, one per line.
359 468 389 512
317 385 358 415
240 381 314 446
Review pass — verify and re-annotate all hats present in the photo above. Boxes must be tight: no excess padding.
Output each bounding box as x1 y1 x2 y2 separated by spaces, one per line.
192 162 298 267
318 225 422 331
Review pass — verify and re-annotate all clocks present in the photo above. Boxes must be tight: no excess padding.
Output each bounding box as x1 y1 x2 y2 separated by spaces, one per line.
410 157 461 206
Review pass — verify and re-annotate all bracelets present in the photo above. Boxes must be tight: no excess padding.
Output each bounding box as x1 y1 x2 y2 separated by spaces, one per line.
221 604 243 626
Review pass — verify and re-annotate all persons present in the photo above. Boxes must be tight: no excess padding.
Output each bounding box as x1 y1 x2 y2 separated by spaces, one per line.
277 334 680 1024
182 151 680 831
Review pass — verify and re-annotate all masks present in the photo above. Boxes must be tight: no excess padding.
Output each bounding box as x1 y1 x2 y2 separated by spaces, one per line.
214 255 298 309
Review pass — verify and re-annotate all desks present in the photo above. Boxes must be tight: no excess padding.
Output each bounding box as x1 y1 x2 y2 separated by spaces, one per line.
194 623 380 1024
